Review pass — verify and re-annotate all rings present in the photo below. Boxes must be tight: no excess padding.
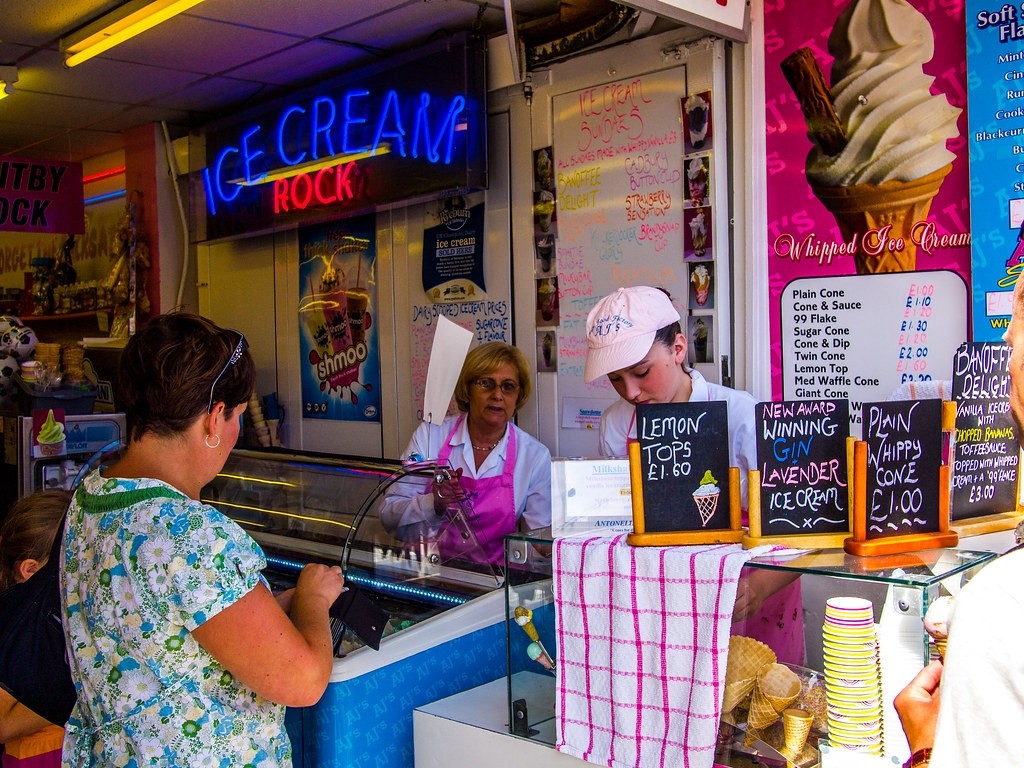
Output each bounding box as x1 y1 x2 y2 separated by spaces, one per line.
438 491 446 499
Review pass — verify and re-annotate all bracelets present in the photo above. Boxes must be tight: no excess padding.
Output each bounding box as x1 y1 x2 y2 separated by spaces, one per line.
902 747 932 768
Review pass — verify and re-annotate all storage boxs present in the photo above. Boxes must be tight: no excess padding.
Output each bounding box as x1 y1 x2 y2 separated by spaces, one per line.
12 369 98 415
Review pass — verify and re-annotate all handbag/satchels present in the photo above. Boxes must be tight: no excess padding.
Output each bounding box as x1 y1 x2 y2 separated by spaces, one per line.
0 465 77 728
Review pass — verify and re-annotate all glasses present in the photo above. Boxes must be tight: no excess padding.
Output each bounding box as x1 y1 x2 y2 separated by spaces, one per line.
473 375 520 397
207 329 250 415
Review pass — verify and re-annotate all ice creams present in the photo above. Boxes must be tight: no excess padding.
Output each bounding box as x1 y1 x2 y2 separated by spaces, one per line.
514 606 539 641
924 596 955 662
780 0 962 276
527 643 552 670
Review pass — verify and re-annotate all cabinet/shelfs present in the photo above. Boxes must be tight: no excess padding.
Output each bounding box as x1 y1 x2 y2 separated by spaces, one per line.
411 532 1024 768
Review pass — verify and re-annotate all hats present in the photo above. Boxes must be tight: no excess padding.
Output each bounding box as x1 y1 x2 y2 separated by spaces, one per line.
583 286 680 384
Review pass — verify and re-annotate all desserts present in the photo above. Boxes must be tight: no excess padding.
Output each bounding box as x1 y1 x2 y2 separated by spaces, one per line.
38 409 64 442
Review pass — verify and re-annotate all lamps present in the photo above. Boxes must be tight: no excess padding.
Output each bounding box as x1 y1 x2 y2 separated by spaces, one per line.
227 142 390 187
0 65 19 101
63 0 207 70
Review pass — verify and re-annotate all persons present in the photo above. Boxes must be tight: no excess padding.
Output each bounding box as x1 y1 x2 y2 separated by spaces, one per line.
0 489 74 768
892 267 1024 768
583 285 822 667
378 341 556 571
60 313 344 768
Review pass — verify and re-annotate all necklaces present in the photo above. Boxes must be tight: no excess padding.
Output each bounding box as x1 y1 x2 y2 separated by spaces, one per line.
473 439 501 451
